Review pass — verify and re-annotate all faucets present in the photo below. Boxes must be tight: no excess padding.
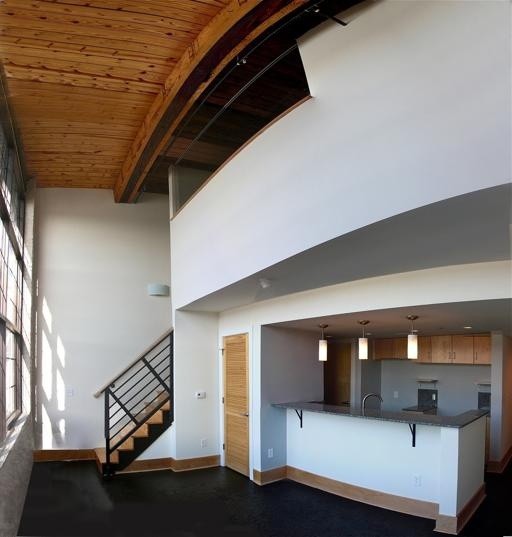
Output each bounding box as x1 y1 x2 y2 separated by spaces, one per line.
361 391 384 415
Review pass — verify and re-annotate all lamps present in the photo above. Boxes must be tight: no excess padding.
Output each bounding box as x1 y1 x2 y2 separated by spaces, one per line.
147 284 169 296
407 315 419 359
358 320 370 359
317 322 329 362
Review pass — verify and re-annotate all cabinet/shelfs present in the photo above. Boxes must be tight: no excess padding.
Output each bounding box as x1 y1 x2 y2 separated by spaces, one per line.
371 335 431 363
431 335 474 365
474 336 490 365
